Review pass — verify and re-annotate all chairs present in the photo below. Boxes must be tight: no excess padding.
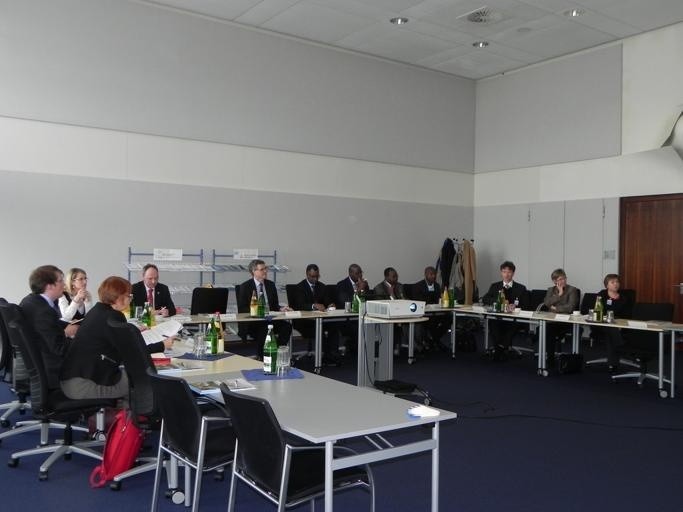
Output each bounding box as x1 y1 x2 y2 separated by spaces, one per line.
108 324 226 492
1 298 119 483
148 372 235 511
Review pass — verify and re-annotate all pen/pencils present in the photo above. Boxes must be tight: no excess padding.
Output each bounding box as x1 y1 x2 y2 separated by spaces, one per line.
162 335 181 341
159 306 166 310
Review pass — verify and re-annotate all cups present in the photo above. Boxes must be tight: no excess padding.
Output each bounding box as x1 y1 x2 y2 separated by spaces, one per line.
572 310 580 320
276 345 290 377
263 304 269 317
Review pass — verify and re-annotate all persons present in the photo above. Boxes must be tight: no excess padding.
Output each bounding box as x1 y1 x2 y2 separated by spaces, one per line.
336 264 370 352
17 265 80 387
414 266 451 352
129 265 176 318
483 262 528 360
292 264 344 370
373 268 423 356
60 274 152 451
56 268 93 323
593 274 631 372
531 269 581 361
237 260 291 358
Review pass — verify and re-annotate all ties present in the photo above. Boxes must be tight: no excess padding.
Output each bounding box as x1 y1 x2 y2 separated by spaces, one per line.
311 286 316 297
147 288 154 308
259 283 265 305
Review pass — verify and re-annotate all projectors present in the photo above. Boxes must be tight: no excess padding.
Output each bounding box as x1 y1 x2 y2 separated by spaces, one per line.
366 300 426 319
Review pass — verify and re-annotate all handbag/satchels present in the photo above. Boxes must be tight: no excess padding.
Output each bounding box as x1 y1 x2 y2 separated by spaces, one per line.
89 409 145 489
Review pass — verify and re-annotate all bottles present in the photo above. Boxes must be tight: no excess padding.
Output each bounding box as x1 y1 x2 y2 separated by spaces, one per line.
194 311 224 360
351 290 359 312
127 293 153 330
258 291 264 317
359 289 364 302
249 288 258 317
592 296 614 323
437 285 456 308
345 301 351 312
262 324 277 376
490 290 516 314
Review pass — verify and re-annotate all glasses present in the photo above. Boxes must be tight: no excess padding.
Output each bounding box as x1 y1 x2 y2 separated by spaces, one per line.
555 276 567 281
254 266 267 272
72 276 87 281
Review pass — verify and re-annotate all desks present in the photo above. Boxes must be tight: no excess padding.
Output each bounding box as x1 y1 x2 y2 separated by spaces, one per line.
183 305 458 373
459 289 683 400
167 339 457 511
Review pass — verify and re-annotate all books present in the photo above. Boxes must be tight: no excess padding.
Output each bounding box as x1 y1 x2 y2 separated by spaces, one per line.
154 361 205 374
140 319 183 345
188 378 255 395
